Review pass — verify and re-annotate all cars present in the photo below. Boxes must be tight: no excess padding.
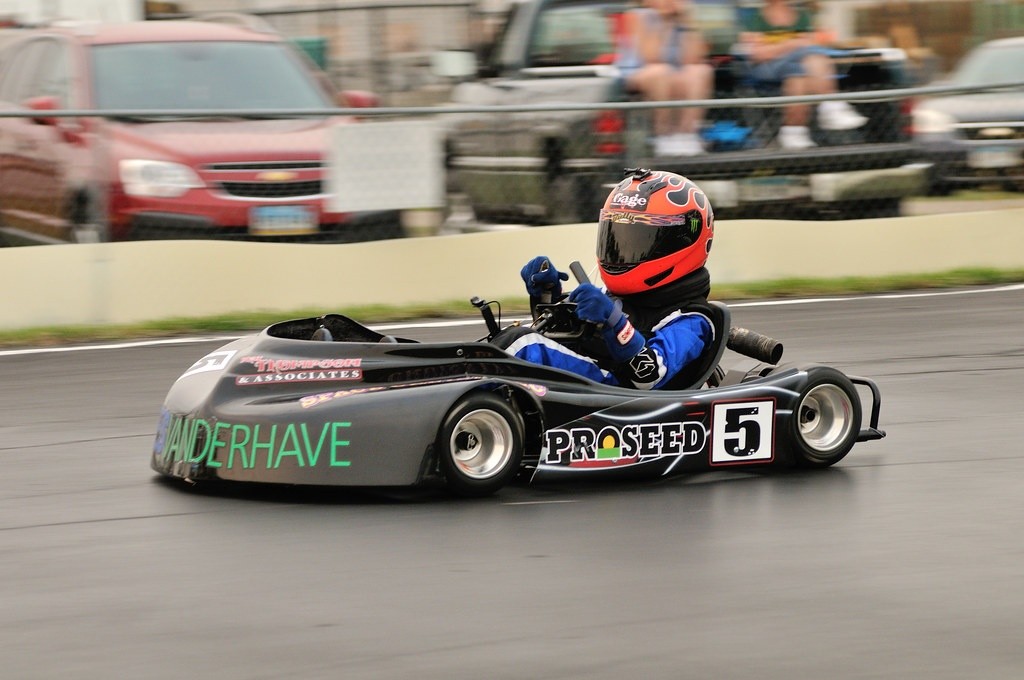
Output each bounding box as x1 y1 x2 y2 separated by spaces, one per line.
3 14 418 247
910 36 1024 197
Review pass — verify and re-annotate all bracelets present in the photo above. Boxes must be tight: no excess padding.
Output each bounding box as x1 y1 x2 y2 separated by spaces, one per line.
679 27 696 32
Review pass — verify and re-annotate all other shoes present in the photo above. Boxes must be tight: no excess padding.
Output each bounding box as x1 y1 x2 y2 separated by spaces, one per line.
778 126 815 152
819 102 868 130
651 132 704 159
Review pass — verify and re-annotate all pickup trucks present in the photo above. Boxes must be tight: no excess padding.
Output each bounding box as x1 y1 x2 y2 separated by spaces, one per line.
438 1 936 226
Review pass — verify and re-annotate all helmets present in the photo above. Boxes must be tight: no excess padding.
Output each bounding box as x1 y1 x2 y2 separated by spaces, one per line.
597 166 716 315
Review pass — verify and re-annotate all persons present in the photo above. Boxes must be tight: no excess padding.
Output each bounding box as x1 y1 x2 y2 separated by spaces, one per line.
614 0 713 159
307 171 716 390
738 0 870 148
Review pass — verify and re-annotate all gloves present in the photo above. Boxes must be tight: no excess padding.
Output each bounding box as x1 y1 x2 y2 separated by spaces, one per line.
569 283 646 364
521 256 562 302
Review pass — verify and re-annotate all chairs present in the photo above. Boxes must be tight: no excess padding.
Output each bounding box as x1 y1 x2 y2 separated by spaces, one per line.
667 301 732 390
